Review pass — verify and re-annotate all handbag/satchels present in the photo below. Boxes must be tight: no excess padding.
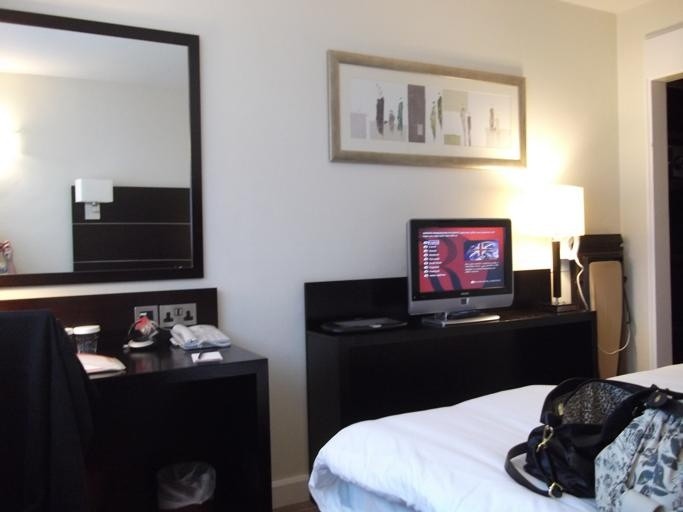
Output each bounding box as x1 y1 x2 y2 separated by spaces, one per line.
505 376 683 512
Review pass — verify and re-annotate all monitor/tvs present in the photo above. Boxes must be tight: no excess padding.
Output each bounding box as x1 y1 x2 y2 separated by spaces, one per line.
405 217 515 327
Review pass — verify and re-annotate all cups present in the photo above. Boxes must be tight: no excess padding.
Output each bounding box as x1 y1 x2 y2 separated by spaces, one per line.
73 326 100 354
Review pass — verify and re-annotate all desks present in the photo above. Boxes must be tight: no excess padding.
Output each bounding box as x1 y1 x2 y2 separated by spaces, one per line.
1 289 274 511
301 268 597 509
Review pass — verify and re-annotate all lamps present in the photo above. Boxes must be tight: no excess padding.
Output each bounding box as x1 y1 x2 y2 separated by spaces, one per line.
74 177 116 221
536 185 586 315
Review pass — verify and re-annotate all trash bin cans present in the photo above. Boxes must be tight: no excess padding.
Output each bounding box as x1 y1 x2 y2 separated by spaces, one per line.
156 460 217 512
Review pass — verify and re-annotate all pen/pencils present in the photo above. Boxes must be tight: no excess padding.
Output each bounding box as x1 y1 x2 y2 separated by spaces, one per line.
198 350 204 358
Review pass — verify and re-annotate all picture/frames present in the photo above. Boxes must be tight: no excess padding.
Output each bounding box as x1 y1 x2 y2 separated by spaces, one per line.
328 50 530 170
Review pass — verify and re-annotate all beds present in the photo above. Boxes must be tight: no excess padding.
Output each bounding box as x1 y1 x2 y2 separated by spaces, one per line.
318 364 683 512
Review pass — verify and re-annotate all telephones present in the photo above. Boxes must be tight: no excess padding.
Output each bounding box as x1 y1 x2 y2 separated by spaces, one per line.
170 324 232 349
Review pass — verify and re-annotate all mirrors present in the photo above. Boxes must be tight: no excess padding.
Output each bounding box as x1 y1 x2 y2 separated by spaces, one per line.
0 8 206 288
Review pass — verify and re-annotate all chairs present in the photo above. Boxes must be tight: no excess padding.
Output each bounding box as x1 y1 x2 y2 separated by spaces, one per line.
1 308 108 512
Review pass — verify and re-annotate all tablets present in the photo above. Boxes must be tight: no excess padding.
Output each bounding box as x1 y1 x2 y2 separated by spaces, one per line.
322 316 405 332
